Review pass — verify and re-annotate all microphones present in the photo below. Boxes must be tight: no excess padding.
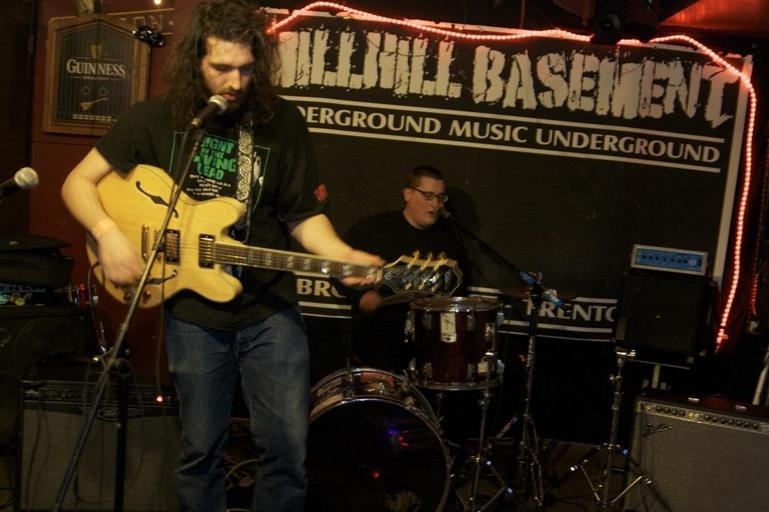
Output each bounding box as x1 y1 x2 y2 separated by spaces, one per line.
0 167 39 205
433 205 451 218
190 94 228 129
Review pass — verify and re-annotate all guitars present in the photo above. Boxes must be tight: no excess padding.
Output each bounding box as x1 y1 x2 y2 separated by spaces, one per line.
86 164 463 308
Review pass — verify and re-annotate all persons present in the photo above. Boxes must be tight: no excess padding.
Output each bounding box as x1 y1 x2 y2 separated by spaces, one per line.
61 0 386 512
330 166 496 380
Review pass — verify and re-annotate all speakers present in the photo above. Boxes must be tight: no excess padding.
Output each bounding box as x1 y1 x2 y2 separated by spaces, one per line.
613 268 717 367
15 380 179 512
0 303 95 396
621 388 769 512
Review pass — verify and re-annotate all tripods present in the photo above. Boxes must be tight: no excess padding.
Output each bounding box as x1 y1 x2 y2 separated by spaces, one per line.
546 350 672 512
450 308 549 512
421 392 471 512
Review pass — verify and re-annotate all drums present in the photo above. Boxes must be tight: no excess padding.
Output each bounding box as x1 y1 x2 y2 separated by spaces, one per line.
405 296 504 389
308 368 452 512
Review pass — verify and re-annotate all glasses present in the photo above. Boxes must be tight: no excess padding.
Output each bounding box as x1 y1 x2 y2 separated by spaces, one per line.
409 186 449 202
